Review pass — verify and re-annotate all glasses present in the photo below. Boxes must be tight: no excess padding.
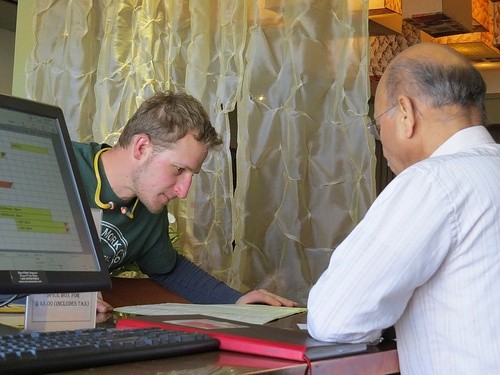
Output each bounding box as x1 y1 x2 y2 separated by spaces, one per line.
365 103 424 140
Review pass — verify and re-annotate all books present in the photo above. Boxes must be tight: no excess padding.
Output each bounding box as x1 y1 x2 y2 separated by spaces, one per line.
116 314 367 374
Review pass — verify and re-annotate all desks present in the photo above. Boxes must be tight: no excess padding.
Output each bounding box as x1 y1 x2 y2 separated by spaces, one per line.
0 278 400 375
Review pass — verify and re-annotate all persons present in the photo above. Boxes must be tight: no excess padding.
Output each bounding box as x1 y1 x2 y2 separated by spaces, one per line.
71 92 300 312
307 40 499 375
